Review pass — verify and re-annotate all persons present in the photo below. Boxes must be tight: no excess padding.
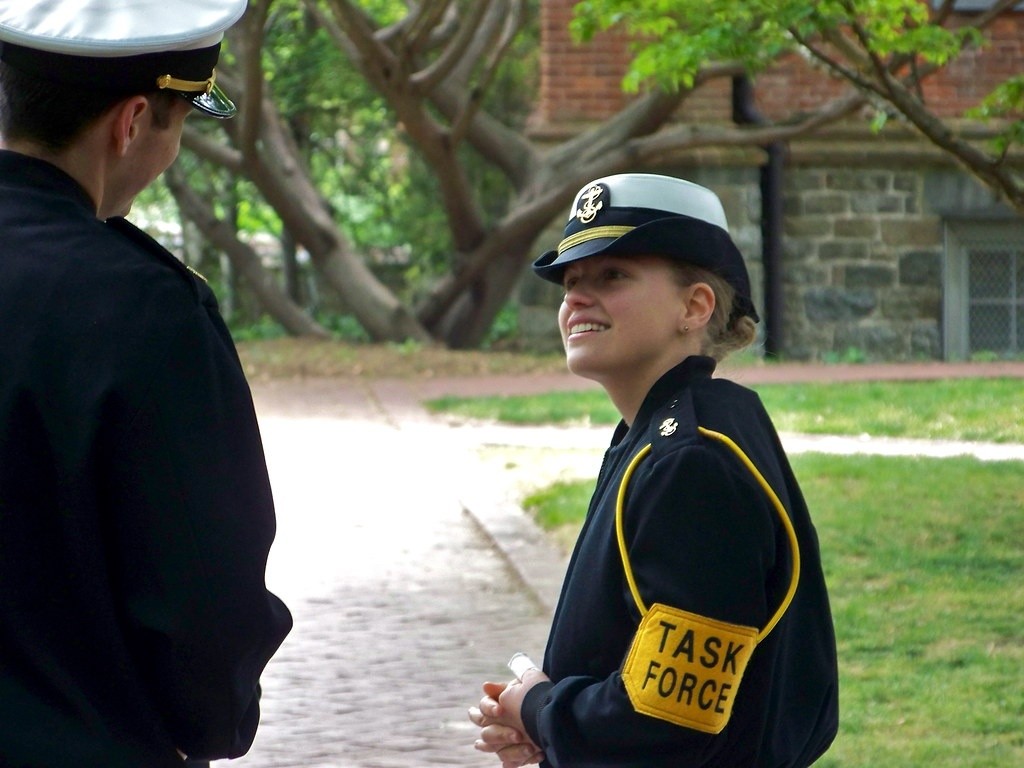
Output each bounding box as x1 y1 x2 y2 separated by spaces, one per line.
465 173 840 768
0 0 295 768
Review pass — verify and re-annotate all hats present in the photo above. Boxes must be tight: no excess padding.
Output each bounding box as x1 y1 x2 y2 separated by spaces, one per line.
1 0 245 117
533 174 759 317
